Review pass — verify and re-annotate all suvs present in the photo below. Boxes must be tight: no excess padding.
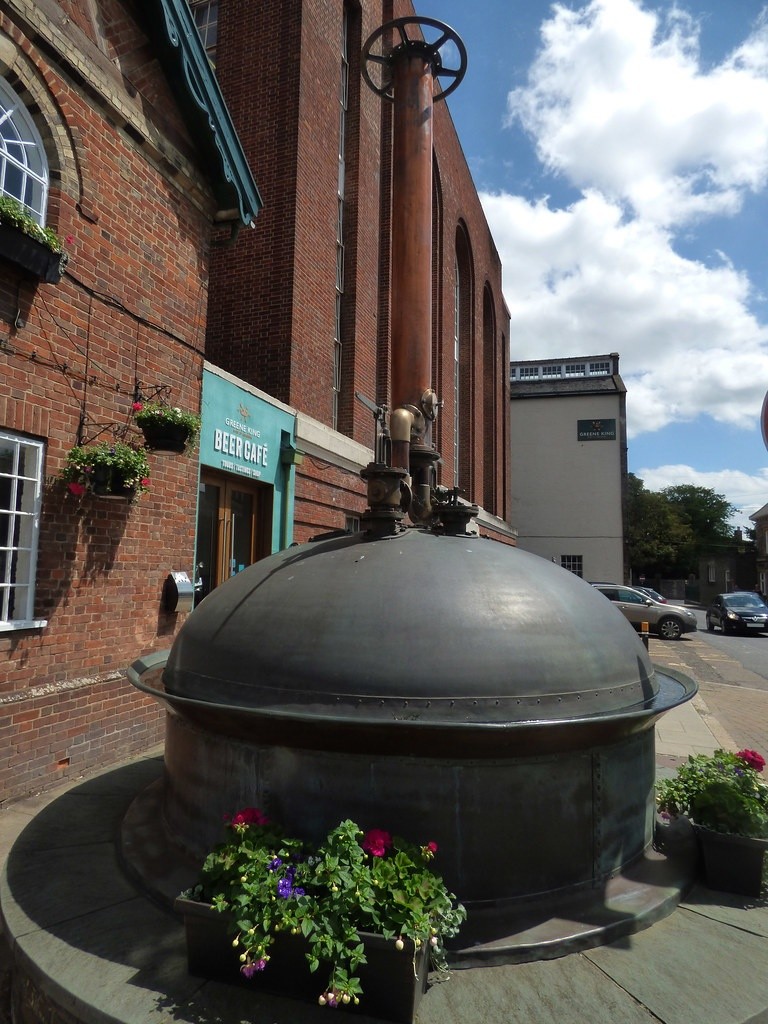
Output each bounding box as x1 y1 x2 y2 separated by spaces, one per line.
588 581 697 640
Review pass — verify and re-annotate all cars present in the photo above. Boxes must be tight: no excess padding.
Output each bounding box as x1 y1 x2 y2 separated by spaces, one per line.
706 592 768 635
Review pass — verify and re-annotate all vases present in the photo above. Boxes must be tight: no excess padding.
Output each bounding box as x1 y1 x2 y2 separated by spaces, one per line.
696 825 768 897
93 467 130 499
175 881 430 1024
0 222 61 283
140 420 189 456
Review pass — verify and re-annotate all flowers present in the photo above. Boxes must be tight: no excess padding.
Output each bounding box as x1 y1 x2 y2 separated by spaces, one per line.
128 399 204 458
0 194 75 253
655 749 768 838
58 439 152 503
189 807 470 1013
